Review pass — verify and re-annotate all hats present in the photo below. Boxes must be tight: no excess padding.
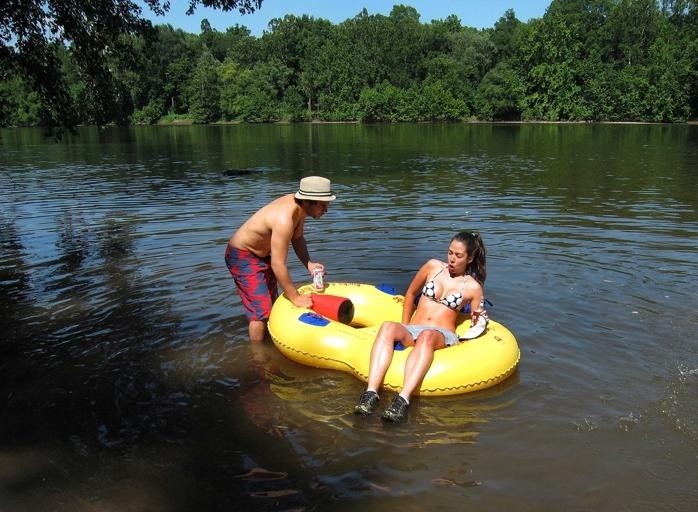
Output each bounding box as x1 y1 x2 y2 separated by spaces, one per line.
295 176 336 201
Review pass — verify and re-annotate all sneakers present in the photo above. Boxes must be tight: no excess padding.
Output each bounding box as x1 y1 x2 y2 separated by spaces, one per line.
354 391 379 417
382 393 409 423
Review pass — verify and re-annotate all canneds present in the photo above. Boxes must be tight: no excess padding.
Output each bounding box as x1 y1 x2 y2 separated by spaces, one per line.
471 310 480 328
312 266 324 292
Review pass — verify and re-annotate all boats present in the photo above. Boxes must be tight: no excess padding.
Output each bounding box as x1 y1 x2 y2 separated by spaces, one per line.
266 281 520 397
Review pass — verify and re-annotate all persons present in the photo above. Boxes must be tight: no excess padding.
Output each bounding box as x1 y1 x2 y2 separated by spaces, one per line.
224 174 338 344
353 230 489 424
